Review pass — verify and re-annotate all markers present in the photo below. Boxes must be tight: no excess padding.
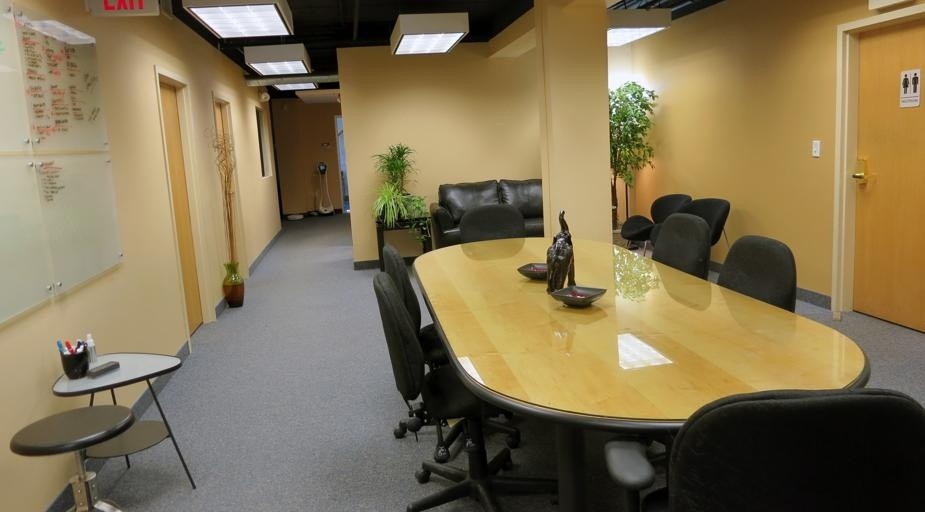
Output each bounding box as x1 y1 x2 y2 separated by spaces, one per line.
56 339 87 355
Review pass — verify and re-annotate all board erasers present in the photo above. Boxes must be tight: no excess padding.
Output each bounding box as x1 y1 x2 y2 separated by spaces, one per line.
88 361 119 377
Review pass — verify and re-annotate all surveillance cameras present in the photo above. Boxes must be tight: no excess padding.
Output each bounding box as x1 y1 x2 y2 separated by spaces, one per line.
258 86 270 102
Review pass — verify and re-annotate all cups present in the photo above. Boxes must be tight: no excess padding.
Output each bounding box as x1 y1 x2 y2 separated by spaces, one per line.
60 348 89 379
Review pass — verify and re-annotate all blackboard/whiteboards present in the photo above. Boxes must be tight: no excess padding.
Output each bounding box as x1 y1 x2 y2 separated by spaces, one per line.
0 2 122 330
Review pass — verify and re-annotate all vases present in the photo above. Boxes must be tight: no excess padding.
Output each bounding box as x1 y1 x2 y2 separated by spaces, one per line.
224 259 244 307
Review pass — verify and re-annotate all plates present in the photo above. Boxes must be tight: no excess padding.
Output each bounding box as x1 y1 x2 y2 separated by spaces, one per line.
518 263 548 280
553 286 607 308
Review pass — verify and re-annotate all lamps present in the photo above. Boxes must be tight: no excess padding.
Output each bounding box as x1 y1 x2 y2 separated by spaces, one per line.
182 0 295 40
605 0 674 47
391 0 468 55
272 83 320 93
244 43 312 76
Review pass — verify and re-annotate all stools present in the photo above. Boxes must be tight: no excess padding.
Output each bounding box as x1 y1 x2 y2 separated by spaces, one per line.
9 406 135 512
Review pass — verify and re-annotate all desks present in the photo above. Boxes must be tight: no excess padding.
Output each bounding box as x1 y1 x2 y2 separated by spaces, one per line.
51 351 196 490
